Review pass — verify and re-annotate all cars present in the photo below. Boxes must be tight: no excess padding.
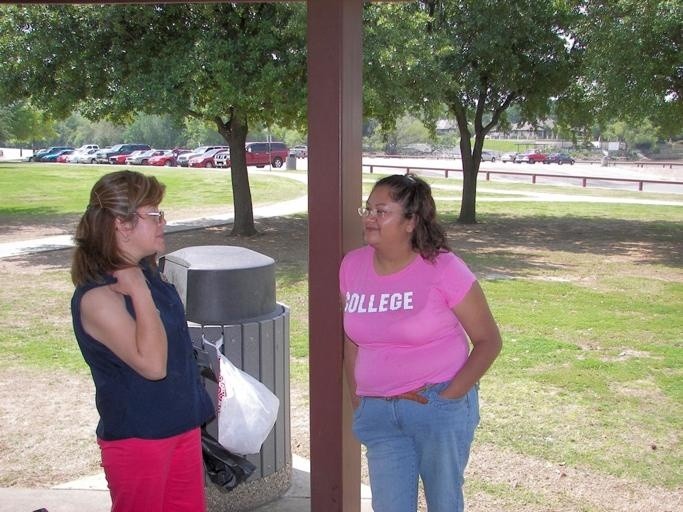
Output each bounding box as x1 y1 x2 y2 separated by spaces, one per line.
31 143 217 167
478 150 575 165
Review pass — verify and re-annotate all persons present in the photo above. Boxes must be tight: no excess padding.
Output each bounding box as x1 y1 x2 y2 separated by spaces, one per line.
69 170 216 511
337 172 504 511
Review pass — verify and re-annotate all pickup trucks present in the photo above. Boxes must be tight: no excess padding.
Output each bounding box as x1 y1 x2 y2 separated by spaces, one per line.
289 145 308 159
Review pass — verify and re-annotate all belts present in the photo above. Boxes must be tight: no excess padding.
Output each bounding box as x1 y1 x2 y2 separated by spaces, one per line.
383 387 428 404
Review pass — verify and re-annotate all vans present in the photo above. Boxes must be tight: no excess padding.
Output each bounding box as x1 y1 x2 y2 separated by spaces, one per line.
213 141 288 167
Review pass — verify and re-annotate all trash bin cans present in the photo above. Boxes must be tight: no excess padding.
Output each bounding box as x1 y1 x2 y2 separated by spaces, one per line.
159 246 292 512
286 154 296 170
601 156 608 166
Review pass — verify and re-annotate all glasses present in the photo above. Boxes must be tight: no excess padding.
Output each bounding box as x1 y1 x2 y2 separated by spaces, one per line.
130 210 165 222
357 207 394 218
